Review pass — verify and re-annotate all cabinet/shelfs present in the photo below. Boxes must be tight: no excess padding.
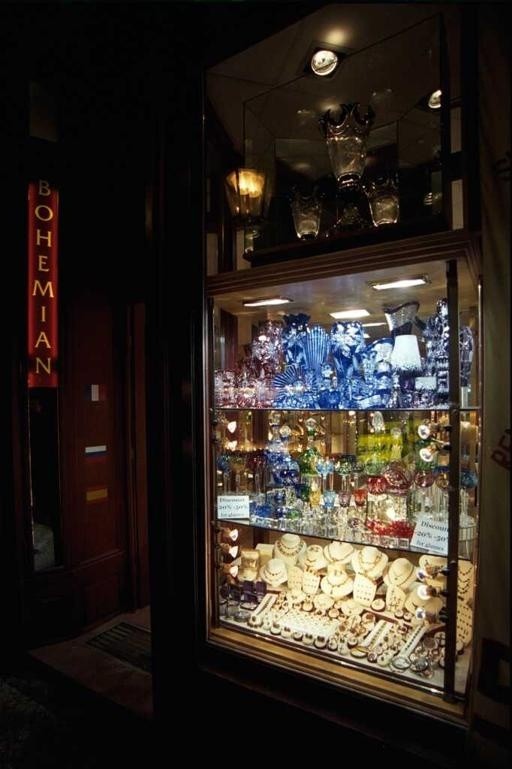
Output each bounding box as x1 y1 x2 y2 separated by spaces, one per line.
1 289 150 651
194 225 485 734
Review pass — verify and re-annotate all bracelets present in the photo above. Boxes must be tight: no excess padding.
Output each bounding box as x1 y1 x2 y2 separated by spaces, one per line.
366 647 446 683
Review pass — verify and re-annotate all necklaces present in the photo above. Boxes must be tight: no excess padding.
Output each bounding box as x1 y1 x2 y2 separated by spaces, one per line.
457 566 475 604
247 533 451 645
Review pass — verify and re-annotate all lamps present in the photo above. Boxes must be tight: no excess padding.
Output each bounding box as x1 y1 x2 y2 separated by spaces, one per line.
227 168 274 239
